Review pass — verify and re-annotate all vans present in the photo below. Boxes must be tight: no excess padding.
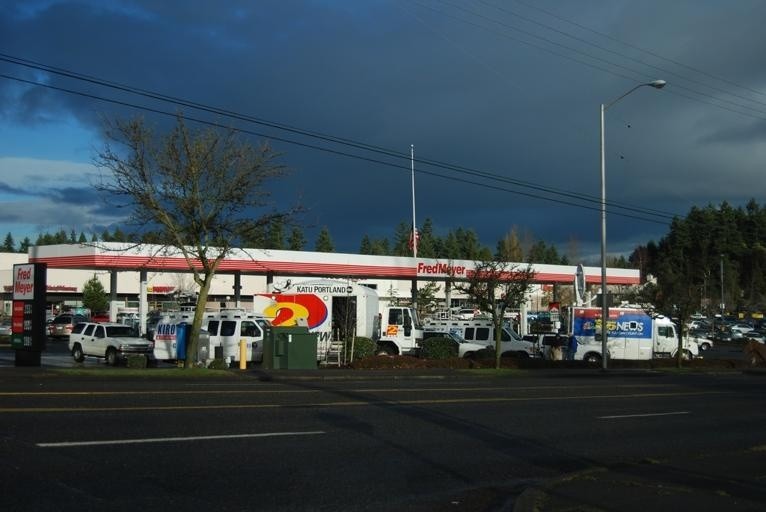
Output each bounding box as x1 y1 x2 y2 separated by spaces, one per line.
153 312 271 367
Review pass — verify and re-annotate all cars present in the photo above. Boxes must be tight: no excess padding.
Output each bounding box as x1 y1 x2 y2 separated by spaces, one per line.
0 314 154 366
687 313 766 351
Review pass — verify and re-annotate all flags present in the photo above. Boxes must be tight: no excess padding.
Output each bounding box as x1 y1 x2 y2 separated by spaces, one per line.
409 225 421 252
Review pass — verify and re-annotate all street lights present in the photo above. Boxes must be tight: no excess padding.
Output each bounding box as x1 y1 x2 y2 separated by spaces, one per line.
600 80 666 369
